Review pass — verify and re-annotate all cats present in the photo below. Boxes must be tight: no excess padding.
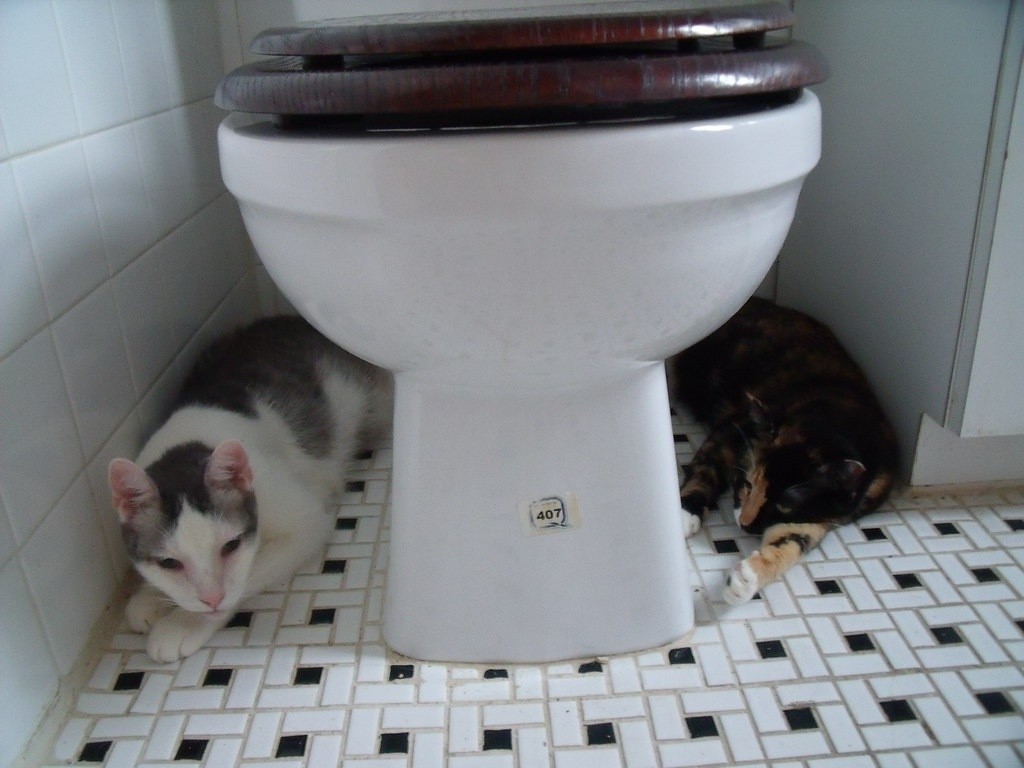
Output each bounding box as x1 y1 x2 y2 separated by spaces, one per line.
676 293 899 606
106 310 383 663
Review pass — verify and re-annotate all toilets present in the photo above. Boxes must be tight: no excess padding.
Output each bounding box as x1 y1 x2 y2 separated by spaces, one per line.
209 12 827 669
